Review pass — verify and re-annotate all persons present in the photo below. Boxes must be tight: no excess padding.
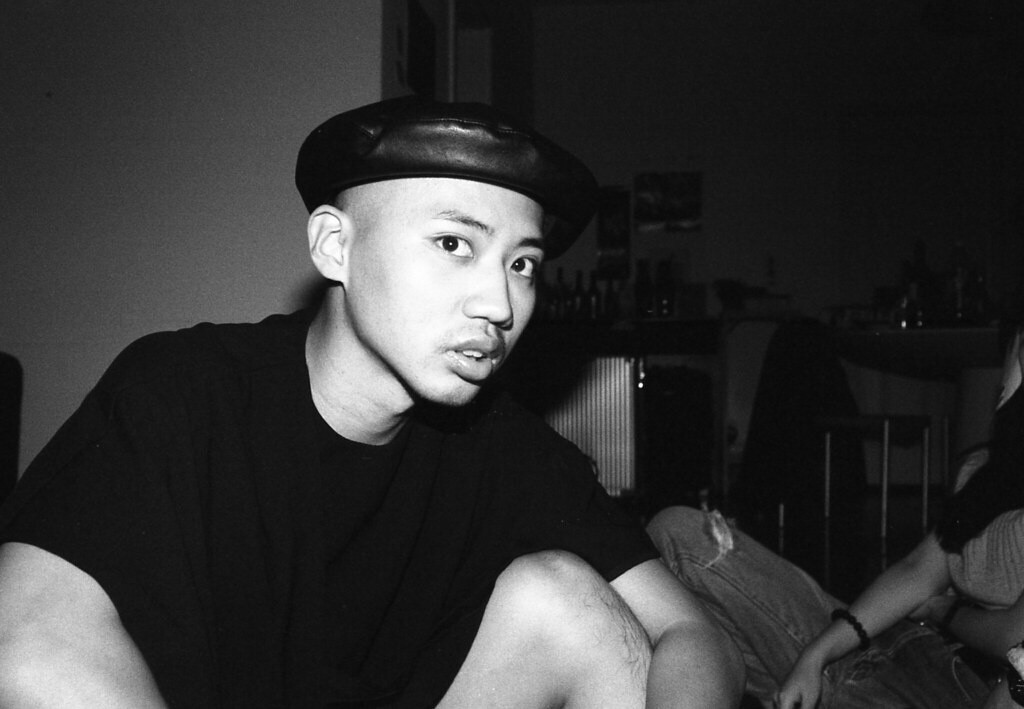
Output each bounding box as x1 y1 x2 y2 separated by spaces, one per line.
645 329 1023 709
0 89 745 709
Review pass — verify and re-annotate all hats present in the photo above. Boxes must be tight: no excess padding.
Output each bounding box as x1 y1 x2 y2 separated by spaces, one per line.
295 96 601 262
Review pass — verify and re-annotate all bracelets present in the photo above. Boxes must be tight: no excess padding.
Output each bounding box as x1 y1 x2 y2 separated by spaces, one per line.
831 608 870 649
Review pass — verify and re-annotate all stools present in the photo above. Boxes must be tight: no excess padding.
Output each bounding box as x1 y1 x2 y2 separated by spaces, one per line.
764 405 938 537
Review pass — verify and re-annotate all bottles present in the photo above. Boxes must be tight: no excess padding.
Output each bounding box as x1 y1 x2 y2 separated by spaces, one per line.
945 273 976 328
573 270 587 321
553 267 572 323
587 270 601 320
604 271 619 316
656 259 673 317
892 299 908 331
907 281 927 328
636 258 653 316
533 278 552 320
